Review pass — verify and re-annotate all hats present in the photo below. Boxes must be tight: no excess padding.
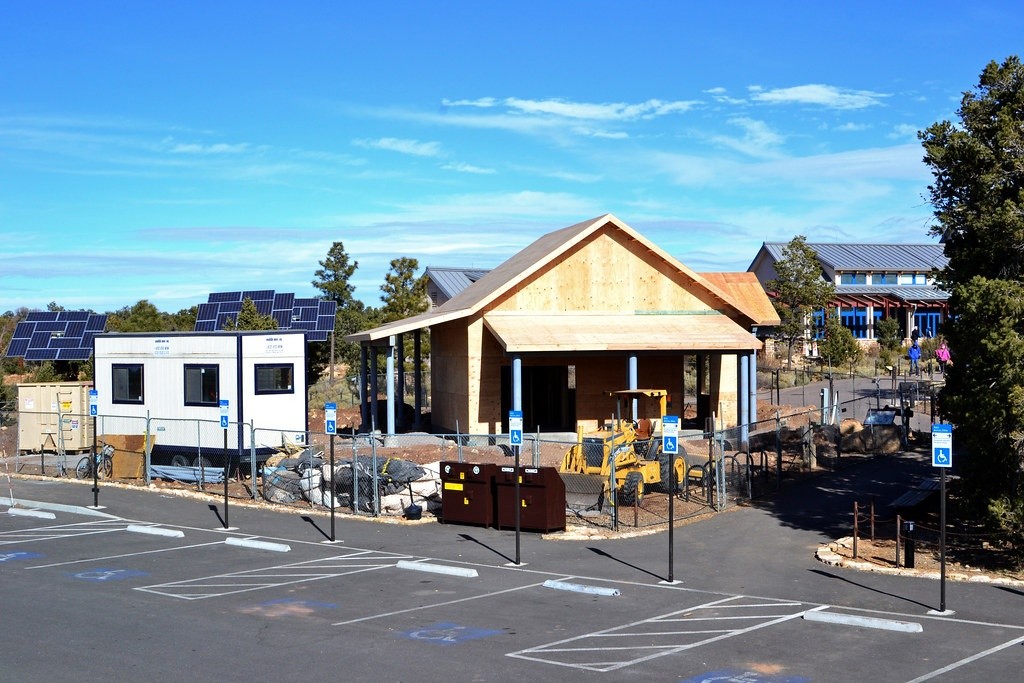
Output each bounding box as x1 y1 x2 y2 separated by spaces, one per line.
941 344 945 348
914 341 918 346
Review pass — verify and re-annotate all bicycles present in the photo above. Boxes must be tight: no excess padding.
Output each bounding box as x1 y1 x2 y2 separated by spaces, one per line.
76 440 115 480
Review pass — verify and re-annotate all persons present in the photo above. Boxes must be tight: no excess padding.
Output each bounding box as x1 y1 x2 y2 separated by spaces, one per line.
912 326 921 343
936 344 950 373
908 342 920 377
899 329 904 345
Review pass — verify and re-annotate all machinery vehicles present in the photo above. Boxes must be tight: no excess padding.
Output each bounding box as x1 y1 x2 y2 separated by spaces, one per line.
558 390 689 516
